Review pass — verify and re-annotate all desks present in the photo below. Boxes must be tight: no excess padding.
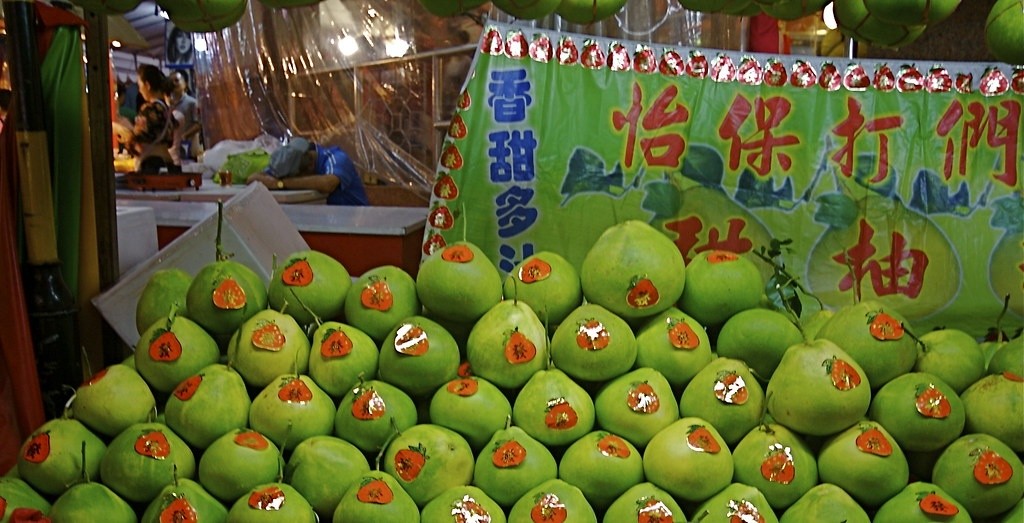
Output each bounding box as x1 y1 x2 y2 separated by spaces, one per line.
120 198 429 283
114 173 329 204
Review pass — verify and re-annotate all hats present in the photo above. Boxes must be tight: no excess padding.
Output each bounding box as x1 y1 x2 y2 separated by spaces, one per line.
270 138 309 178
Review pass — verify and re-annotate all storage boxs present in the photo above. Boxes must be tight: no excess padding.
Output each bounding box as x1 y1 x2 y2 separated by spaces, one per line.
91 182 312 352
115 204 160 276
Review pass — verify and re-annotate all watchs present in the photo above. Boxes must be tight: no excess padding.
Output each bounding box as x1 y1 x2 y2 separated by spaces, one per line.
275 178 284 190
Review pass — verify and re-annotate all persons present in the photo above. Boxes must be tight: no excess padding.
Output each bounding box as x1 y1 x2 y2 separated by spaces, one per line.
118 63 202 174
247 136 367 206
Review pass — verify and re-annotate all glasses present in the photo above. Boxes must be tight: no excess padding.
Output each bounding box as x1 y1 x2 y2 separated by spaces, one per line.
170 78 183 84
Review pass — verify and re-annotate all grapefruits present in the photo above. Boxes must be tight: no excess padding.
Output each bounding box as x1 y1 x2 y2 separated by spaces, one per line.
805 199 961 314
648 185 775 263
989 228 1024 322
0 222 1024 523
